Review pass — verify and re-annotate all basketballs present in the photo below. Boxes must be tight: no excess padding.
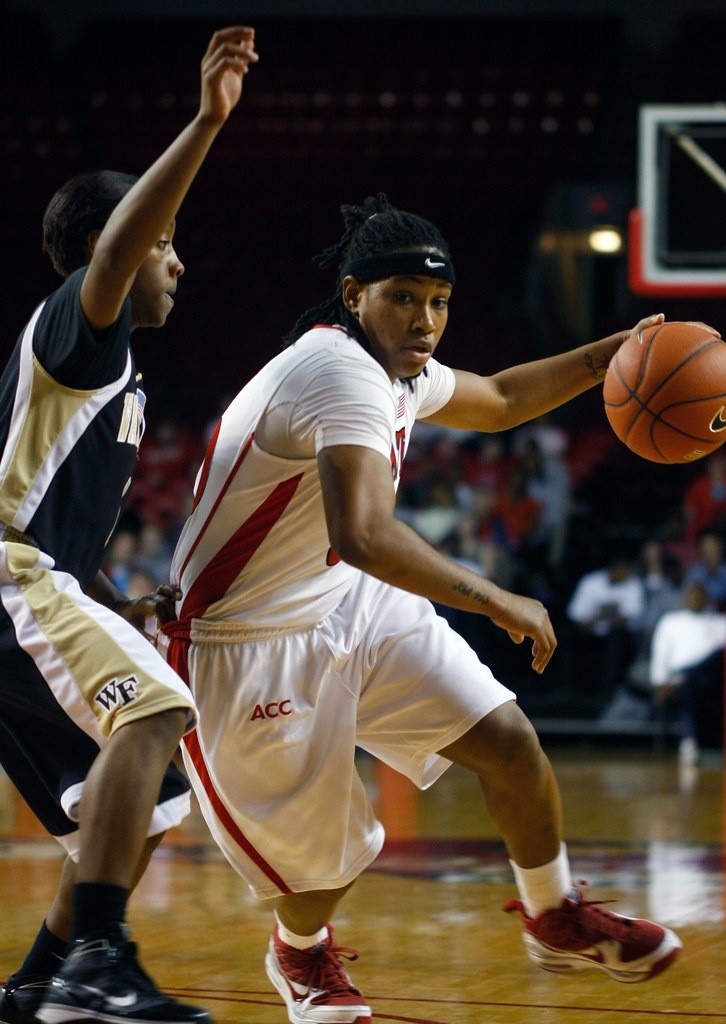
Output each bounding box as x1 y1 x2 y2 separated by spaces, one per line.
601 322 726 465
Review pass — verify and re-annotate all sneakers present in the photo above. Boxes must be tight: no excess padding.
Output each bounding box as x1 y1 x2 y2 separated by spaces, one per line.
0 973 52 1024
265 922 372 1024
501 879 683 983
34 923 211 1024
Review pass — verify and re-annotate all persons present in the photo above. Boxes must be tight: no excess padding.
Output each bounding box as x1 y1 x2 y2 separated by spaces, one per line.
154 214 684 1023
83 408 726 759
0 27 259 1024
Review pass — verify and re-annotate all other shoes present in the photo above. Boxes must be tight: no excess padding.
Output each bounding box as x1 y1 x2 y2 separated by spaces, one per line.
678 745 702 766
